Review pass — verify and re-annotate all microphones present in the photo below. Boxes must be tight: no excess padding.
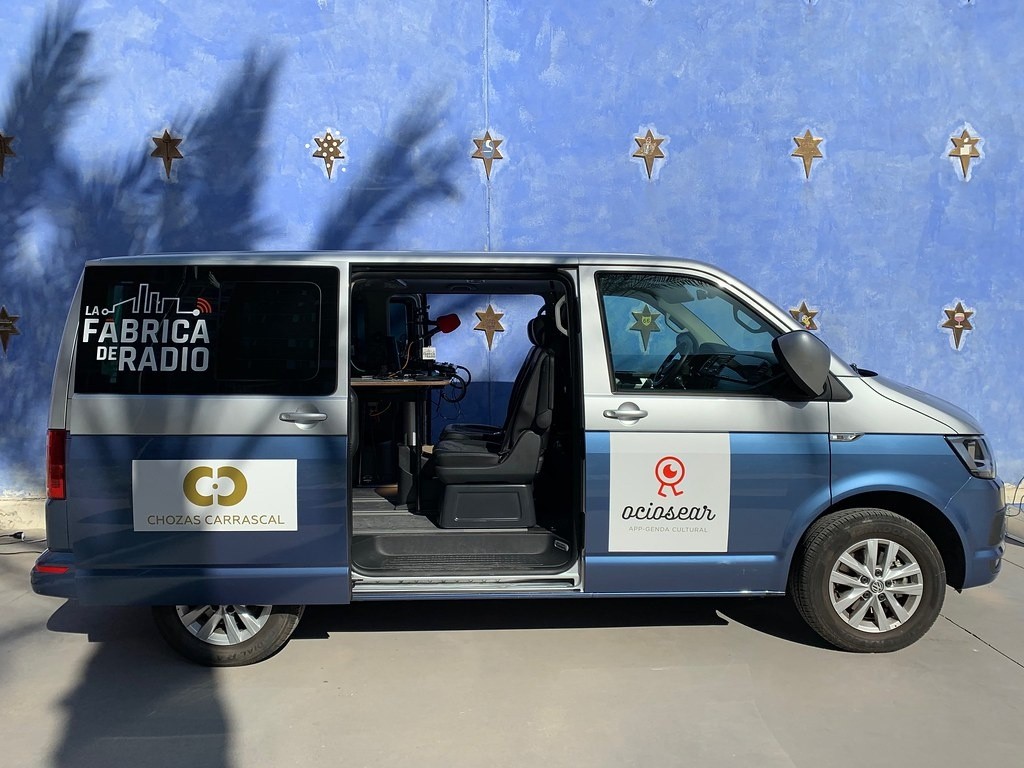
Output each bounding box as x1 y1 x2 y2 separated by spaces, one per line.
410 313 461 344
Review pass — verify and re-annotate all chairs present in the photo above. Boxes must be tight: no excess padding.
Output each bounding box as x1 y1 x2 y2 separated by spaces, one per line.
431 315 552 484
438 318 534 444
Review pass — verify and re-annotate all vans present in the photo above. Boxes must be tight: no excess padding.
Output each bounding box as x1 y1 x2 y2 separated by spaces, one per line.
29 247 1009 669
